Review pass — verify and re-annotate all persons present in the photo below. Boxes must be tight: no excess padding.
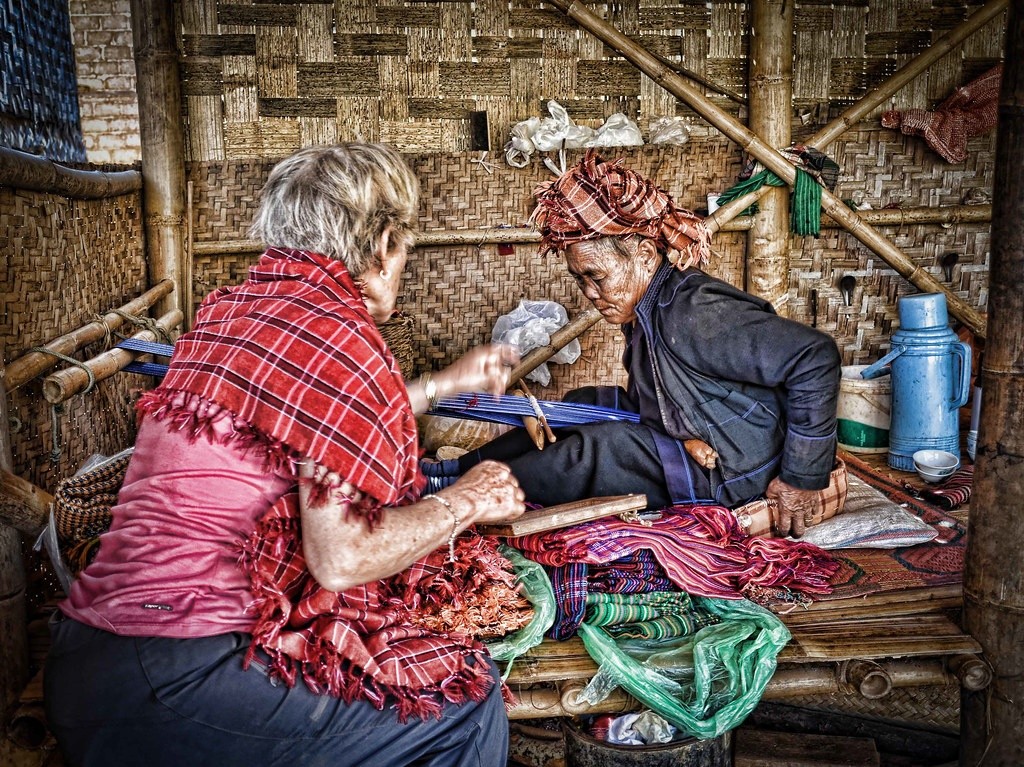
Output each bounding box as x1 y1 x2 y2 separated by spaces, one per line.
38 138 529 767
417 147 845 539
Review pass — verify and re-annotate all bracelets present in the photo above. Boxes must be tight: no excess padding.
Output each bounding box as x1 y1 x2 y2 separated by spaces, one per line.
418 369 440 416
414 489 463 567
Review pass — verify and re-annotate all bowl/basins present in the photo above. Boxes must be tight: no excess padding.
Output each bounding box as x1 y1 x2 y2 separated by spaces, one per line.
912 450 957 484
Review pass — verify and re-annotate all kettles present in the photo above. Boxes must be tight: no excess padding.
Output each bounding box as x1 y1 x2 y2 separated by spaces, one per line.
860 292 972 470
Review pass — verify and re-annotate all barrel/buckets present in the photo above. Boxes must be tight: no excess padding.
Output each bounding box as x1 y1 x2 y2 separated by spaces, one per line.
838 363 895 457
